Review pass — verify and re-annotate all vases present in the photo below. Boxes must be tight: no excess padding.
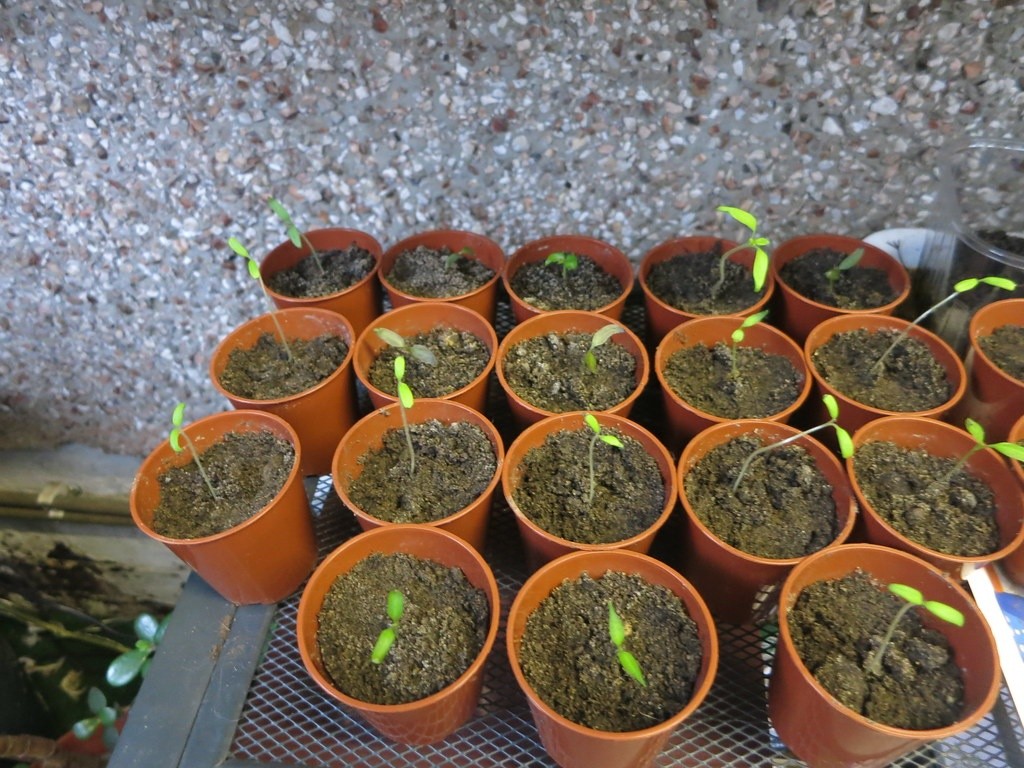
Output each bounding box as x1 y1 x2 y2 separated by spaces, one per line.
969 297 1024 435
999 417 1024 586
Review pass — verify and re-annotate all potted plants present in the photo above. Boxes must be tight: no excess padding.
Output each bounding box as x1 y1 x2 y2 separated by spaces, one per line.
38 609 178 768
129 135 1023 768
